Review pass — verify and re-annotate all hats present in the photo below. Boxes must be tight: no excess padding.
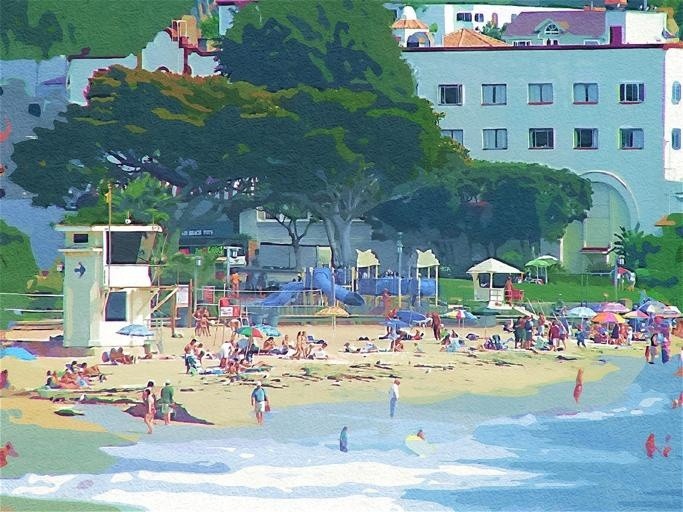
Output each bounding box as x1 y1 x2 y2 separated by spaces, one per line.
256 381 262 386
